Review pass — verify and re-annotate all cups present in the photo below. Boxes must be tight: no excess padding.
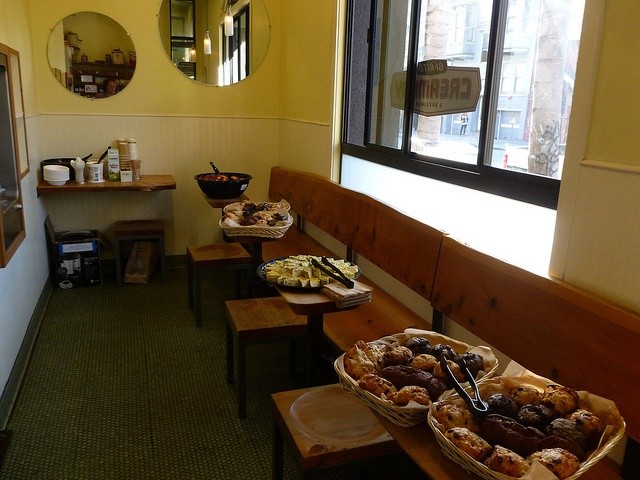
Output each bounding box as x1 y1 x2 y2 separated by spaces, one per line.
131 167 141 180
86 162 104 181
117 137 130 170
128 138 138 160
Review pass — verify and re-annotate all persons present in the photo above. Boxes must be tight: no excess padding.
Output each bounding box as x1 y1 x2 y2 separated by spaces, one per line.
95 80 117 99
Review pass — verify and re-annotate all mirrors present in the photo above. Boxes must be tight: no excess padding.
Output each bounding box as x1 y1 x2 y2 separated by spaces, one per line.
157 1 271 87
172 39 195 81
168 2 193 39
46 10 136 100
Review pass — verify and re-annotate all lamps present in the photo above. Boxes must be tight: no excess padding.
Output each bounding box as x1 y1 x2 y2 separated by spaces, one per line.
204 1 211 54
191 1 196 62
224 1 234 37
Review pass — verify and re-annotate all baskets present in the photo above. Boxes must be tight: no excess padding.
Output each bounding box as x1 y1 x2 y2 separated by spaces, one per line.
334 329 499 428
218 214 294 239
427 375 626 480
223 201 290 214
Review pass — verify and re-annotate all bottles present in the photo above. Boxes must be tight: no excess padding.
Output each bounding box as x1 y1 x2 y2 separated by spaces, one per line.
105 53 111 65
81 52 87 62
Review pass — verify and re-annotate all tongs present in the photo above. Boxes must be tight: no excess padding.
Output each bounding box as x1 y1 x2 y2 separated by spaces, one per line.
310 255 355 289
440 355 488 420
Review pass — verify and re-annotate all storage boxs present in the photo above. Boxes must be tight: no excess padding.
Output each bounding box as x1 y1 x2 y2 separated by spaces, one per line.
80 75 93 82
84 84 98 94
46 218 111 288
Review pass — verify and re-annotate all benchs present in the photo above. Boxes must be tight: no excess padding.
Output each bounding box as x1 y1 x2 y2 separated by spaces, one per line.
374 234 640 480
263 166 441 354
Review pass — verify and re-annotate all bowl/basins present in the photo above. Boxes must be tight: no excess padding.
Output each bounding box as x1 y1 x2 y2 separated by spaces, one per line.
192 172 253 199
44 165 70 186
41 157 83 186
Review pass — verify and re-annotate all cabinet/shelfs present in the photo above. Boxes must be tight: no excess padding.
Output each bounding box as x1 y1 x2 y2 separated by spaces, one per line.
74 64 133 98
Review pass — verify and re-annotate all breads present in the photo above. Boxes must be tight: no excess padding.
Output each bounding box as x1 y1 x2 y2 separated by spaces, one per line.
344 333 494 405
435 381 605 480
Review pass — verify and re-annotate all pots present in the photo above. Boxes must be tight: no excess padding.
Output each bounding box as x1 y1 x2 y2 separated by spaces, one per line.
65 33 80 47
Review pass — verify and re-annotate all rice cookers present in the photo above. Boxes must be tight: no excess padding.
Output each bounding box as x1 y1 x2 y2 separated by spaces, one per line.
111 49 125 65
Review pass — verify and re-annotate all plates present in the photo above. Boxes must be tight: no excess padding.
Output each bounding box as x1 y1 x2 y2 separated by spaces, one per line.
84 180 106 184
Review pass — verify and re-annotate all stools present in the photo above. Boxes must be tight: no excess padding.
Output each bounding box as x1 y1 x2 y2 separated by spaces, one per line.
186 242 251 327
223 296 308 419
271 383 394 479
113 219 165 286
